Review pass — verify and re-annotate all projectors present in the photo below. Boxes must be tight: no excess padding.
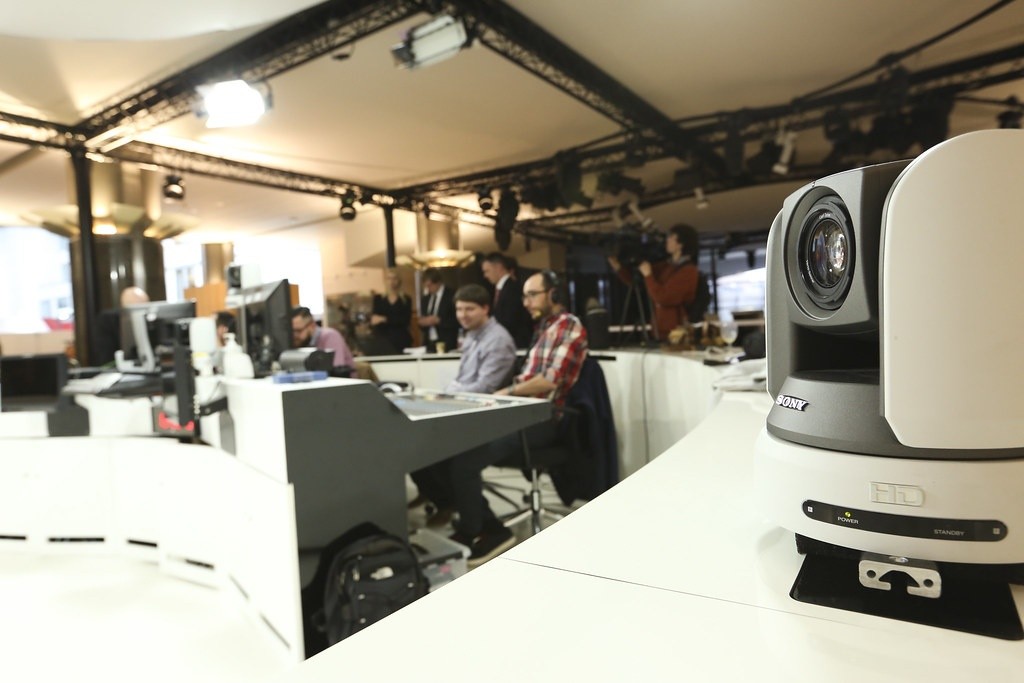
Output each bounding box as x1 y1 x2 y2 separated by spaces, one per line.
388 13 474 73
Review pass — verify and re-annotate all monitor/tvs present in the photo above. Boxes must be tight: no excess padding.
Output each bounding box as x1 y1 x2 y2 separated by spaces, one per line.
118 300 195 376
230 279 292 378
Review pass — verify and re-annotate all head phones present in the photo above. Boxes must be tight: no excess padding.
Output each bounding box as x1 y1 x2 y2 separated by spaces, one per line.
538 267 562 303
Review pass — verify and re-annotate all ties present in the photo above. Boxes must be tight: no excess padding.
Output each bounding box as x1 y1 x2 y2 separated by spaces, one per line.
493 289 499 310
430 295 436 315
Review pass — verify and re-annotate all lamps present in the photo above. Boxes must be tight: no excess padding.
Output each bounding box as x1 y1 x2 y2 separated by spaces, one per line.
478 71 1024 229
339 197 356 218
164 175 184 199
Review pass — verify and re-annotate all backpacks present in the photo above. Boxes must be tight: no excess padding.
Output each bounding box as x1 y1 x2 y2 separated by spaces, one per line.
301 523 432 658
672 260 711 323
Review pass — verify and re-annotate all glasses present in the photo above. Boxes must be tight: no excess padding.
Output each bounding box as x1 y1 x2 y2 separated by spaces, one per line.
291 321 312 334
521 289 548 301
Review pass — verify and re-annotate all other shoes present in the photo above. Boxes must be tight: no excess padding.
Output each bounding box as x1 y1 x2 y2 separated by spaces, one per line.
466 527 517 566
448 527 479 544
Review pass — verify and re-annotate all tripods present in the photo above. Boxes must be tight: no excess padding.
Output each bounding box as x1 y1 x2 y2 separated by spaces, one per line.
615 286 660 347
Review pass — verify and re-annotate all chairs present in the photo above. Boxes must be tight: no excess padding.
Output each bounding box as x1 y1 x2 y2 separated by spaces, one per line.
484 350 616 537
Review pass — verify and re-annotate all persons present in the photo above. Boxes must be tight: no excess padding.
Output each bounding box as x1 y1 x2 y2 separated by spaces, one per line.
608 224 711 349
217 251 587 570
